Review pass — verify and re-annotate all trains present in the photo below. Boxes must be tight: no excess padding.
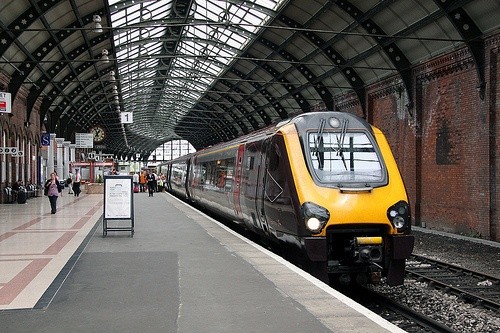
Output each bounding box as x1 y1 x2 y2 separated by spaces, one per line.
151 111 415 288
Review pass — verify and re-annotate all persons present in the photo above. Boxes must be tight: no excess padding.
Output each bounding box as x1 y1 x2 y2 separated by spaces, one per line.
44 171 62 215
12 178 22 191
107 169 120 175
68 168 82 197
133 169 166 197
